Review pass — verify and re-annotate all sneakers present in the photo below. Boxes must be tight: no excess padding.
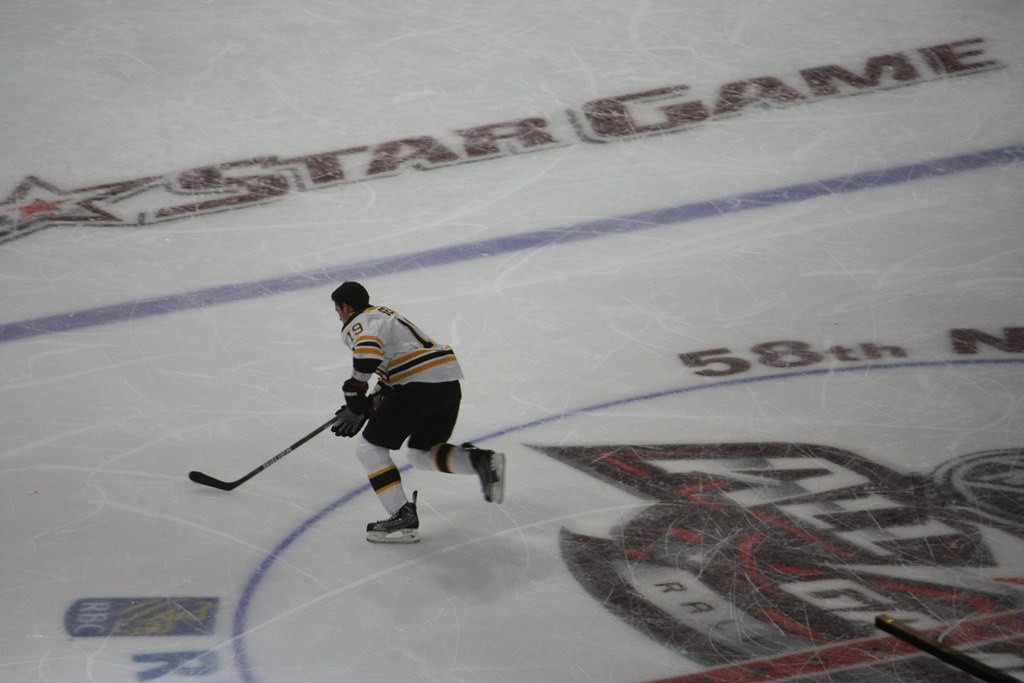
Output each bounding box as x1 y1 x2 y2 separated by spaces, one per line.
461 443 505 503
365 490 421 543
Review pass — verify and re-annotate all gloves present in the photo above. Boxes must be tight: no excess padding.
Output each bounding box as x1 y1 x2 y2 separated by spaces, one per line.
342 377 369 416
331 405 371 438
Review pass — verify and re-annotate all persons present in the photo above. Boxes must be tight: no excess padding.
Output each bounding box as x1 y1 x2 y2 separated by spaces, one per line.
331 280 505 545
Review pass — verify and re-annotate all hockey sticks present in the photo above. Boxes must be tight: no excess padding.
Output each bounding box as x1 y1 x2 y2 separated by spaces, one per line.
188 406 352 492
